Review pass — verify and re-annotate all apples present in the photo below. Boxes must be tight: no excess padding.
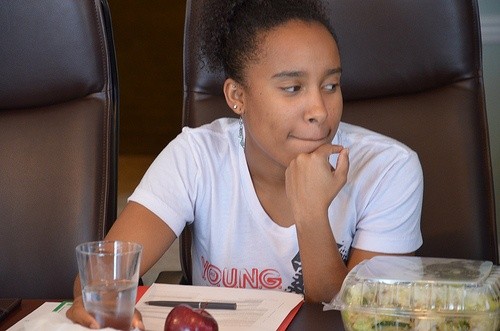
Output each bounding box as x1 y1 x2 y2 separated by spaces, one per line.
164 301 218 331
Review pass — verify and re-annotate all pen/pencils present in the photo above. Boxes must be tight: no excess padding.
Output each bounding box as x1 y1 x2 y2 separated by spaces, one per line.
145 301 236 310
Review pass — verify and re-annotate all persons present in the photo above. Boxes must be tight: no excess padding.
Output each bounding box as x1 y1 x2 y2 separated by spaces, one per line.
66 0 424 331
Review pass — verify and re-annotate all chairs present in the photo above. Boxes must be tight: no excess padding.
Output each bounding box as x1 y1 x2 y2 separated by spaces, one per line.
178 0 500 286
1 0 119 301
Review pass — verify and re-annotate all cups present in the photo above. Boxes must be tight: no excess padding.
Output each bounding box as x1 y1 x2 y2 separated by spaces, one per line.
75 240 143 331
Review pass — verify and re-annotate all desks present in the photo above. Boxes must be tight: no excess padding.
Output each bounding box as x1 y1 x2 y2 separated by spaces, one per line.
0 296 345 331
